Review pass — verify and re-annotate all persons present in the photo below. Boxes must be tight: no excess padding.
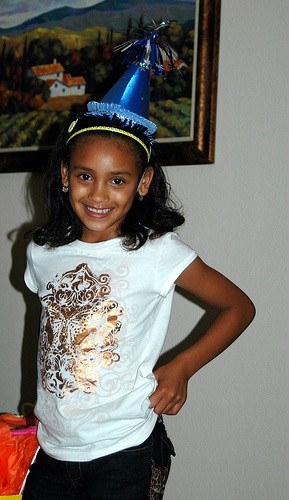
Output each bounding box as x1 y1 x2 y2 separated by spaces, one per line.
22 21 256 500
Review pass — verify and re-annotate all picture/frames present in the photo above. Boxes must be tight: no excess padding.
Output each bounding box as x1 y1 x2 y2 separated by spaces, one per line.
0 0 222 175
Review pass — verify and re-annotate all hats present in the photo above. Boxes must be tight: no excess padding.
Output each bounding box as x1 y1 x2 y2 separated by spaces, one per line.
82 18 181 144
